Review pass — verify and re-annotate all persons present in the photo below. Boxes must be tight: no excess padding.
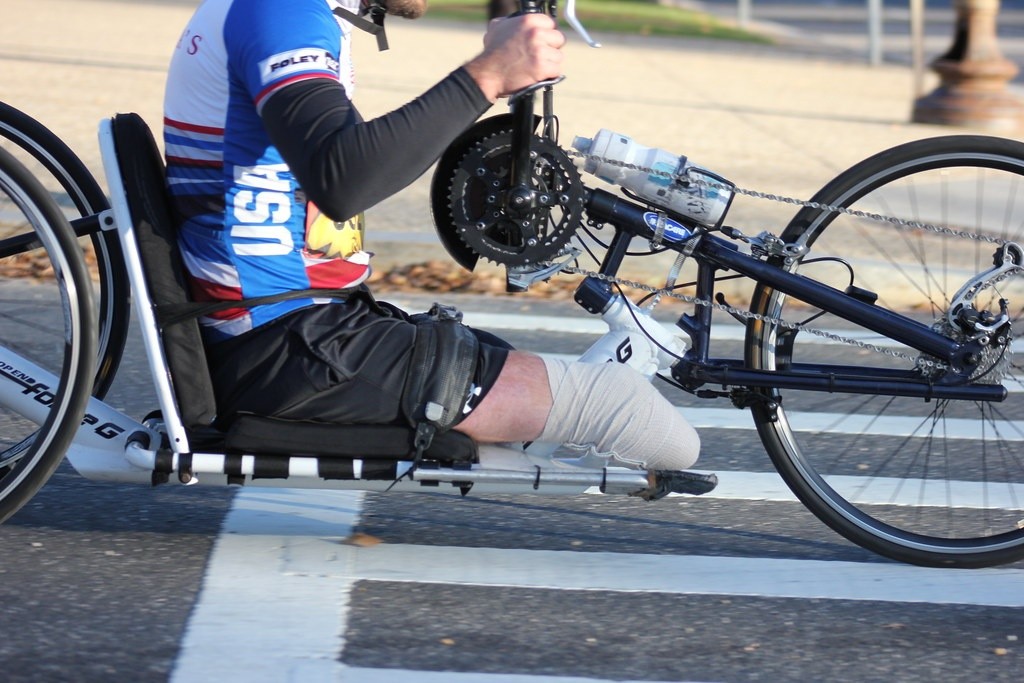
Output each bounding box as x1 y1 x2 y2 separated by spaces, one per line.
161 0 701 471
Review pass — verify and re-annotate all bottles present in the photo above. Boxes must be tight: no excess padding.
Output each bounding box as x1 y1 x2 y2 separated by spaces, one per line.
571 128 731 224
